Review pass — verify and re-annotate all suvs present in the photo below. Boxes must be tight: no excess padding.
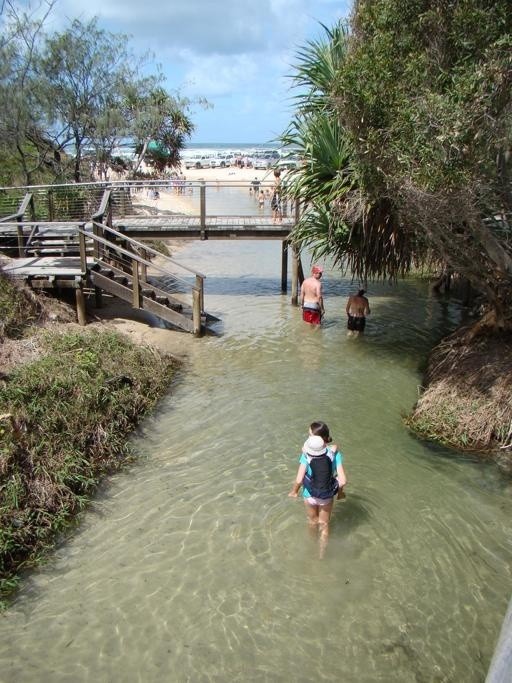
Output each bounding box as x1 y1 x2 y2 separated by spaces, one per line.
184 148 308 173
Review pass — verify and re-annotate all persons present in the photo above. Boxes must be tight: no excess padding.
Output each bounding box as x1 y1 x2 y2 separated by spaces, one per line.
249 170 284 225
136 170 196 198
287 421 347 560
287 435 345 500
300 266 326 329
345 284 371 341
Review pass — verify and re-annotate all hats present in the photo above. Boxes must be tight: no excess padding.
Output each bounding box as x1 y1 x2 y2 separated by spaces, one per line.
359 286 367 292
304 436 327 456
311 265 323 273
